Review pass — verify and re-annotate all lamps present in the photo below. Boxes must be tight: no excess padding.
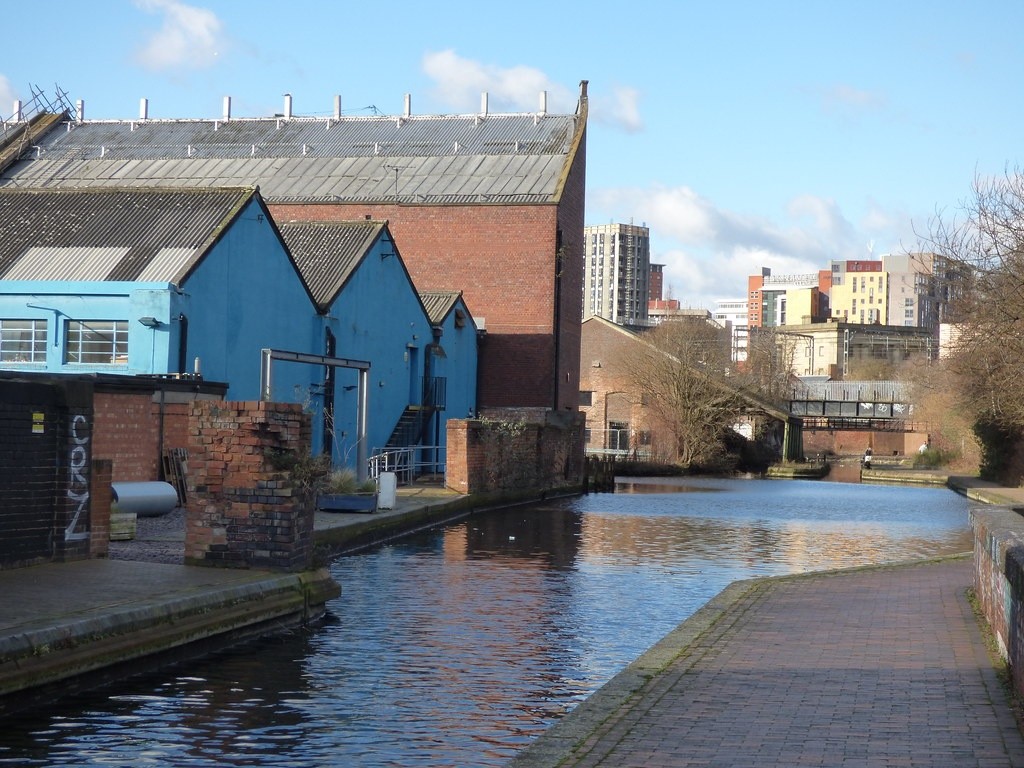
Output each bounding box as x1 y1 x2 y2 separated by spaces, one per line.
138 316 159 329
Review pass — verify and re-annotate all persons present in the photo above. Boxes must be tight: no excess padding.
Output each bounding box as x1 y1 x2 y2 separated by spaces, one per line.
919 441 927 453
866 447 872 469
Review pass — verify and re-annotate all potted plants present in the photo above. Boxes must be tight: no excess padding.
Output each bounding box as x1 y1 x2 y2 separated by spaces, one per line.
317 469 378 513
